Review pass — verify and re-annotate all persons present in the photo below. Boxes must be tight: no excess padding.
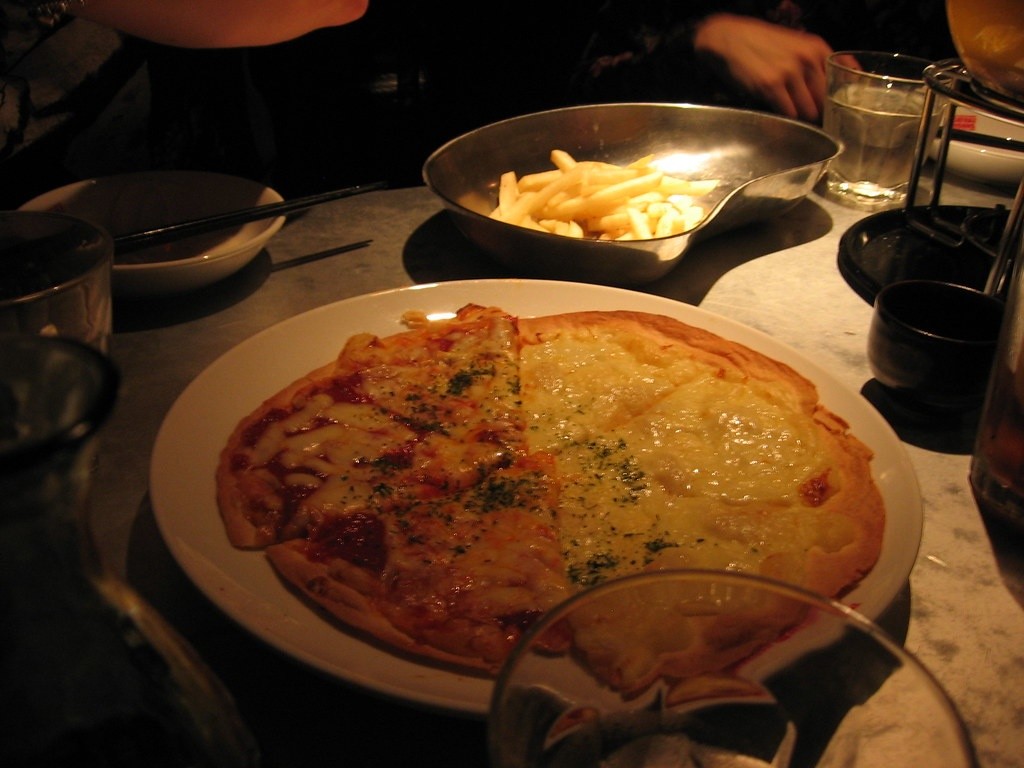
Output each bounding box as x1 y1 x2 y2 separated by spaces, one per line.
0 0 869 210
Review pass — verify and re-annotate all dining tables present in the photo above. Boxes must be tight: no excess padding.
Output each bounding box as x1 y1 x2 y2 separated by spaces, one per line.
75 137 1024 768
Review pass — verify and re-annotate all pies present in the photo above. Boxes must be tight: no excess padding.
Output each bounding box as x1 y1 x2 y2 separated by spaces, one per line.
215 302 888 699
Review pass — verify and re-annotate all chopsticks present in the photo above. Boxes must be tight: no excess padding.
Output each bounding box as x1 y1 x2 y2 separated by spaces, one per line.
113 179 389 255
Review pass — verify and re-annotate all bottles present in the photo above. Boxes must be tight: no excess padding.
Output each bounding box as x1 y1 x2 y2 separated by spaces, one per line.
0 335 260 768
970 225 1023 525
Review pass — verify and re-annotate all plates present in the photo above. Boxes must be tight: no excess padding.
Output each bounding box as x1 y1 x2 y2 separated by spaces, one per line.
14 173 288 299
150 278 925 713
927 105 1024 184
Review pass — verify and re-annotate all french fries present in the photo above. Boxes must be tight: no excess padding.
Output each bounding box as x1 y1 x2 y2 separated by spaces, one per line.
486 150 719 240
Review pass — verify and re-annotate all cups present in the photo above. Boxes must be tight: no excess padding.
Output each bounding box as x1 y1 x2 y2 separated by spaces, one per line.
0 209 111 351
487 569 980 768
824 50 956 207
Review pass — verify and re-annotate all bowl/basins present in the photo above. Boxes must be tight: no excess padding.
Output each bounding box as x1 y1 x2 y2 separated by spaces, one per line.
866 280 1006 427
